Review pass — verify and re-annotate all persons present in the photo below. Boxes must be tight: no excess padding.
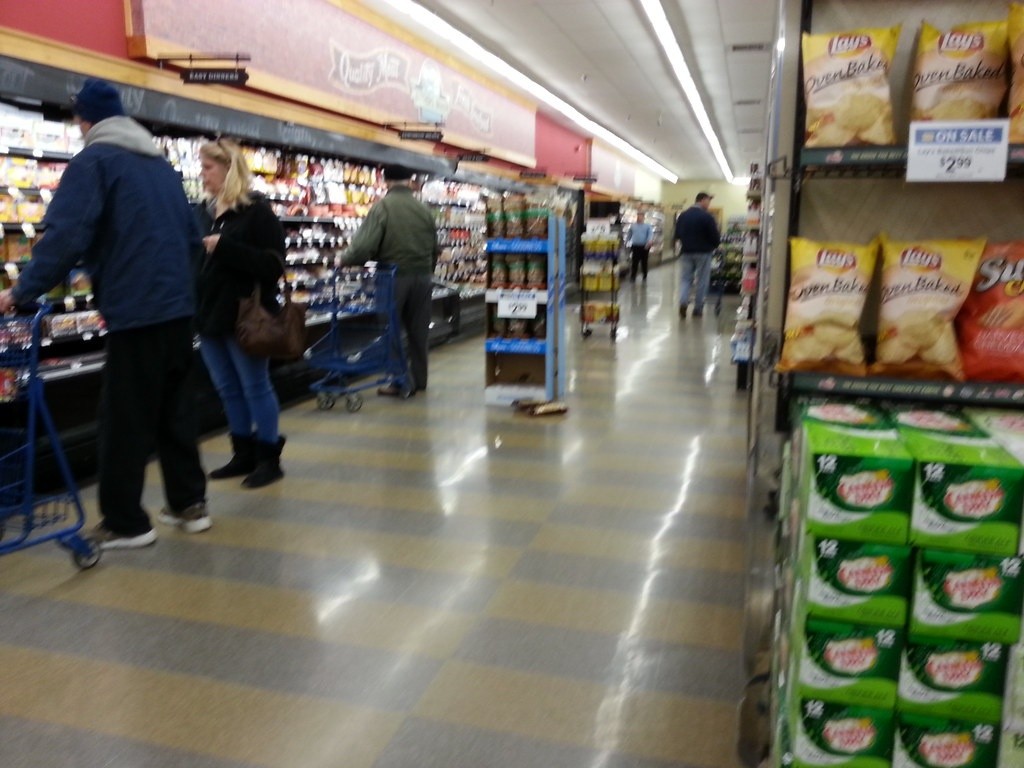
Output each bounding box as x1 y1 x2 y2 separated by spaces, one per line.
341 160 440 398
625 210 655 284
0 77 212 550
187 137 288 487
672 193 722 318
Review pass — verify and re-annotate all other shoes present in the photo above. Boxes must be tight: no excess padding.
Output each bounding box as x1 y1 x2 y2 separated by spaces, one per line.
378 384 427 396
678 304 687 317
691 306 703 318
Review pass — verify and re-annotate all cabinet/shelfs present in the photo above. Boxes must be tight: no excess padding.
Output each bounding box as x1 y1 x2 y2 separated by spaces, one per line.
739 0 1024 588
483 216 565 406
576 239 622 339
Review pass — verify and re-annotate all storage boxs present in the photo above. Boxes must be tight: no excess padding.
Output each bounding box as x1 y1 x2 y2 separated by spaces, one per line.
584 239 618 323
764 394 1024 768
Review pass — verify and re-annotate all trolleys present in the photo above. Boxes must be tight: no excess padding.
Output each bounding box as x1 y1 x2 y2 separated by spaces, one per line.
301 256 413 413
0 300 104 573
582 238 628 341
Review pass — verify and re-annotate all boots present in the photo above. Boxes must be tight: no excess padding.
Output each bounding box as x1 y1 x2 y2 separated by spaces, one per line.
242 429 287 490
210 431 261 479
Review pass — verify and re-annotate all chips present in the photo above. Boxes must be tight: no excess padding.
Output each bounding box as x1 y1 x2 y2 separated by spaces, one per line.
785 91 988 363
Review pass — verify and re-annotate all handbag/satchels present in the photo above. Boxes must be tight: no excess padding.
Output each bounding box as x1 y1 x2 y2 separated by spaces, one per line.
236 249 309 362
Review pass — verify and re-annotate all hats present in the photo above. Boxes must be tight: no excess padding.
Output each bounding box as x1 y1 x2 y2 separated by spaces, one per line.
384 164 415 181
73 77 125 123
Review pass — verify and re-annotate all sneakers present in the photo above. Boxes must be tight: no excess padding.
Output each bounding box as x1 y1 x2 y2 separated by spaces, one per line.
87 518 159 550
156 499 215 534
